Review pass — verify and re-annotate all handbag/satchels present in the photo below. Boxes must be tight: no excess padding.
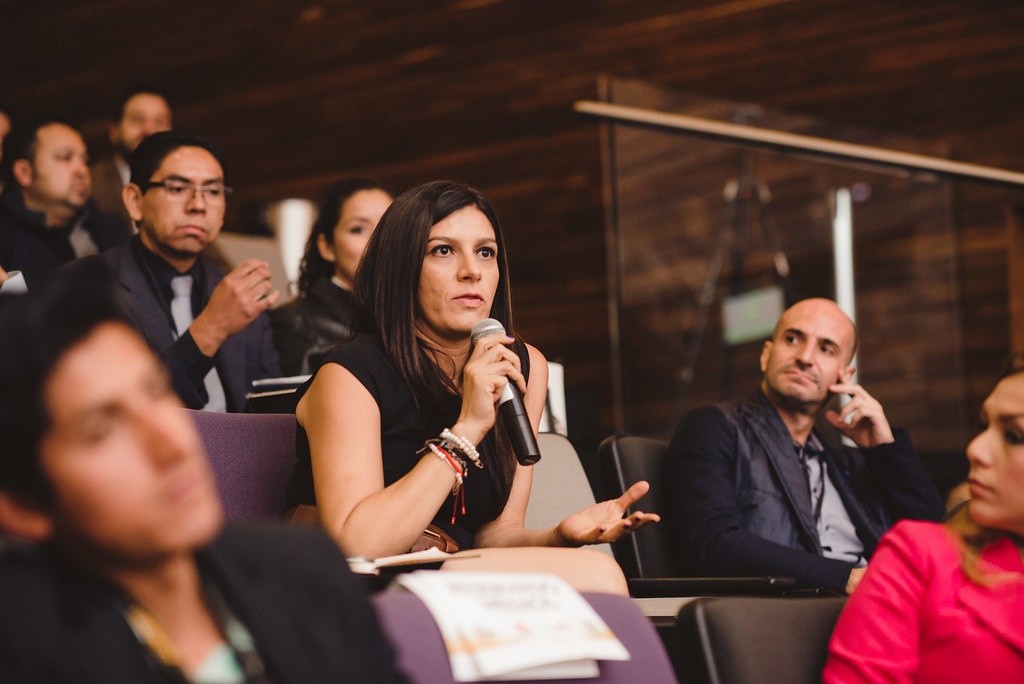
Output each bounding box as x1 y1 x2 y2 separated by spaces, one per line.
289 504 459 552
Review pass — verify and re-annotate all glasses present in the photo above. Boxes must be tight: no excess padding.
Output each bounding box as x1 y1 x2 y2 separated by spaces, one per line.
144 178 233 207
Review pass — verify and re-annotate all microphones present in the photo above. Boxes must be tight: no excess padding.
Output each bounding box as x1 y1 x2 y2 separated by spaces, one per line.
470 319 542 466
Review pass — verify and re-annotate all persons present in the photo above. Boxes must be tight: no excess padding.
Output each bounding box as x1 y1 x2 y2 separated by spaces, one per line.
663 296 1023 684
0 83 395 415
0 287 415 682
292 178 660 597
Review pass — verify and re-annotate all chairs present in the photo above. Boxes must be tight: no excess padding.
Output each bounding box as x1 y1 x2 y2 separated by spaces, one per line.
211 228 301 309
672 596 849 683
523 431 624 559
375 572 677 684
599 435 797 595
177 407 326 542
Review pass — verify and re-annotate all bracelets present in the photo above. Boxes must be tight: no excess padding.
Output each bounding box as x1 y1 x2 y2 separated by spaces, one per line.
415 427 484 526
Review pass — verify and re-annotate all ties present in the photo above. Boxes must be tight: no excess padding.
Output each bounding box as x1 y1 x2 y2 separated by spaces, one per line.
169 276 224 412
